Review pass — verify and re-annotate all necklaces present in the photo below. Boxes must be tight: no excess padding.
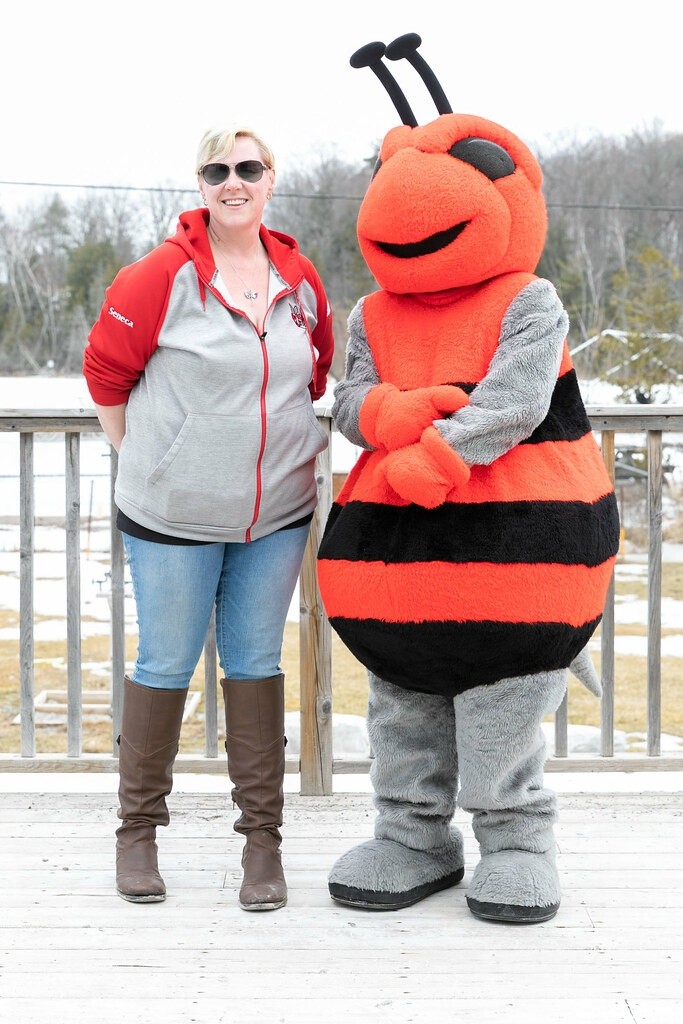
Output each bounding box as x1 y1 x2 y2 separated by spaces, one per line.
208 237 261 305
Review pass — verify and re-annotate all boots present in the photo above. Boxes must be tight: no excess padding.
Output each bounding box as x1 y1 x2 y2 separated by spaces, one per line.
115 672 289 911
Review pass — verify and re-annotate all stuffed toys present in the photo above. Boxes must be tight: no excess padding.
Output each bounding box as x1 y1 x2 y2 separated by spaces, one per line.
317 34 620 923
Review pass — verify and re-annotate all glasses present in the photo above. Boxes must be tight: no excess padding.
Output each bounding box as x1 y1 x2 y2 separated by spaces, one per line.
199 160 270 185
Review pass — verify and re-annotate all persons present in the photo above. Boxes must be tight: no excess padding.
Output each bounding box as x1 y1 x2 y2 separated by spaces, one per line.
81 123 337 912
